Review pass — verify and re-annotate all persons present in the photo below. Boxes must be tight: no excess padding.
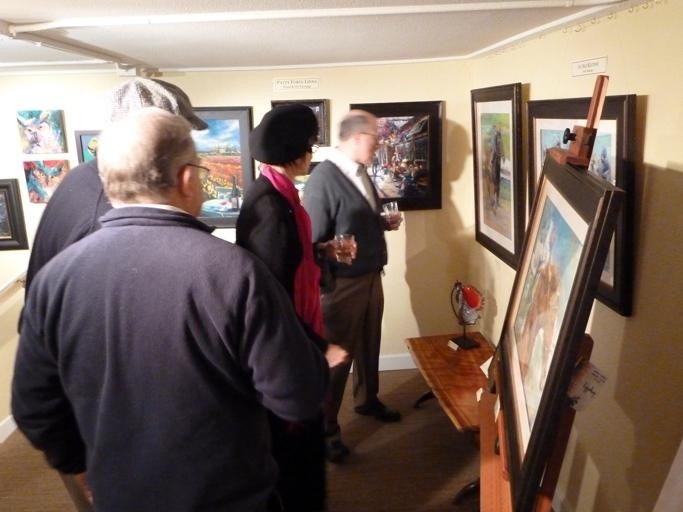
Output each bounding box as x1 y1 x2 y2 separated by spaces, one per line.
236 105 358 512
17 76 209 336
10 107 330 512
303 109 403 463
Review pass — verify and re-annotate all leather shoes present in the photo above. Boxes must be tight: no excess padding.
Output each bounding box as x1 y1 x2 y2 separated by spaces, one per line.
326 441 350 462
354 398 400 422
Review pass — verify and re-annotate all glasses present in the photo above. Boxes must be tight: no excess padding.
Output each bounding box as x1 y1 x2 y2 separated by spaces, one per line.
181 164 210 184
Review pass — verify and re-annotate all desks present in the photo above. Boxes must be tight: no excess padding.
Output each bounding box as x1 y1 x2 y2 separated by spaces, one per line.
453 387 577 512
406 329 495 433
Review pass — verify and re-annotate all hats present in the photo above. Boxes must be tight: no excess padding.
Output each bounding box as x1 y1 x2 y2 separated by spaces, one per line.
250 105 319 166
114 76 208 130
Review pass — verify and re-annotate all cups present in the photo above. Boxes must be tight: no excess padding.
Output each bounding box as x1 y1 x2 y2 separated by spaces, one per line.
334 232 359 264
379 200 402 230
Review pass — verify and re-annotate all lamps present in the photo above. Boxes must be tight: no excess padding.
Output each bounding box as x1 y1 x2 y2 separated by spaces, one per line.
448 280 485 354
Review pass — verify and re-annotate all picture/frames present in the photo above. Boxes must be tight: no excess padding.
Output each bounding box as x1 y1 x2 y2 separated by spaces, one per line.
471 82 525 272
527 94 636 317
75 131 101 168
349 101 443 211
294 162 324 199
191 106 253 229
498 147 626 512
0 179 29 249
271 99 330 147
